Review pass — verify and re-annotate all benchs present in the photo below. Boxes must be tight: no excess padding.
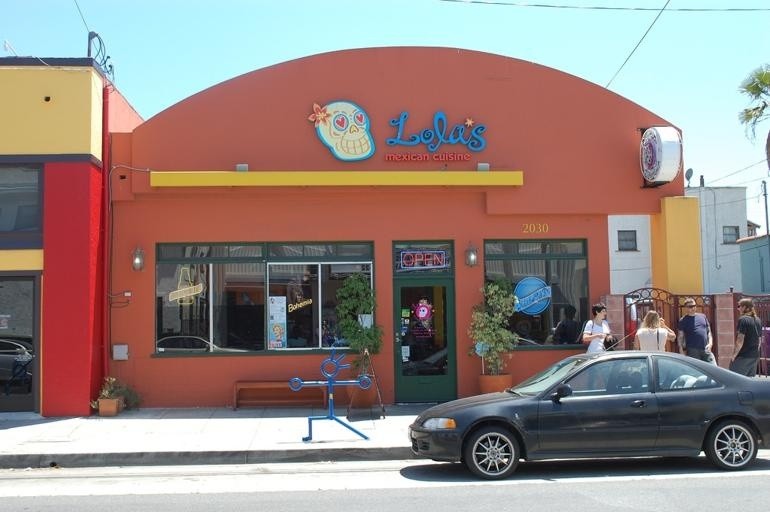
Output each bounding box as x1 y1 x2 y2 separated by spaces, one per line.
234 380 327 410
664 374 708 388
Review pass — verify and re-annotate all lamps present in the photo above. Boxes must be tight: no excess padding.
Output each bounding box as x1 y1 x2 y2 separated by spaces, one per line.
132 247 144 271
465 242 480 267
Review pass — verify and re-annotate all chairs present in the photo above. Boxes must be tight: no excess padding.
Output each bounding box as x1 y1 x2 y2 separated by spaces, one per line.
616 371 642 393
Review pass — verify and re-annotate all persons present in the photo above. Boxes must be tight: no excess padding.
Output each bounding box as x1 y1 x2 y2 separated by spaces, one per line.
674 297 719 366
633 309 677 352
728 297 763 377
551 304 583 345
580 302 614 354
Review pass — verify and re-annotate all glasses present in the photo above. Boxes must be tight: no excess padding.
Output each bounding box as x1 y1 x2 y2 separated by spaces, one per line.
687 305 697 308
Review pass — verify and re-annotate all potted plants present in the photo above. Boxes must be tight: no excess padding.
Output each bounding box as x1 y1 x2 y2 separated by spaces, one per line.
334 273 384 407
468 282 523 393
91 375 143 416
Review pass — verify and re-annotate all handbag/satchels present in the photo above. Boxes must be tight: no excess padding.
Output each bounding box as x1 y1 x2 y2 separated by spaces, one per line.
581 319 594 345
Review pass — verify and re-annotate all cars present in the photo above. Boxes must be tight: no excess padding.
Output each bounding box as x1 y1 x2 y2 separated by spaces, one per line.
0 338 34 383
409 348 770 480
156 335 250 354
